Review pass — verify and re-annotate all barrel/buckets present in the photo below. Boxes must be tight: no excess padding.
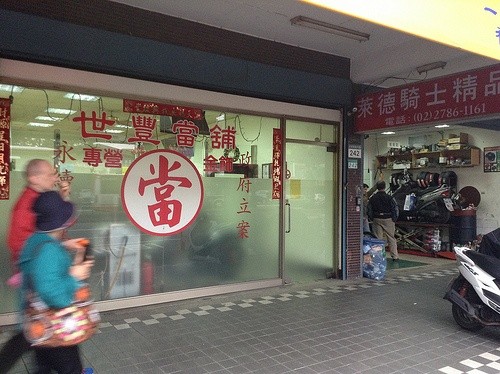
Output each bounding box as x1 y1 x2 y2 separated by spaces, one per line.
448 210 477 253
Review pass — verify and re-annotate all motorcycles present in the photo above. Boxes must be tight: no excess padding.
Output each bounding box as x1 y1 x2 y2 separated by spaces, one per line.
392 168 454 224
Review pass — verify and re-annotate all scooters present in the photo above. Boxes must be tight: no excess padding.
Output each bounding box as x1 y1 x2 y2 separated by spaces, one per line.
442 247 500 332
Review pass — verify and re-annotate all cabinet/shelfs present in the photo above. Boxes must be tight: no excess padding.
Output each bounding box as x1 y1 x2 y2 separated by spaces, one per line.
375 148 481 170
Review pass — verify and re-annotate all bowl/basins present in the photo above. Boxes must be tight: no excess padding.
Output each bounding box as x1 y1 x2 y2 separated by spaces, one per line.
439 157 448 163
420 158 429 166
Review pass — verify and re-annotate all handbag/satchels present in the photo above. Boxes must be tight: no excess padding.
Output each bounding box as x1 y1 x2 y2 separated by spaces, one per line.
21 240 98 347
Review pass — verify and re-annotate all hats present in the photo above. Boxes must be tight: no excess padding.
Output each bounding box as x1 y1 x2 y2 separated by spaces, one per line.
31 191 80 233
377 181 385 186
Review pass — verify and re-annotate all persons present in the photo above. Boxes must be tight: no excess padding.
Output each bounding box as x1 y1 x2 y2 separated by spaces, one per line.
363 179 400 262
0 159 93 374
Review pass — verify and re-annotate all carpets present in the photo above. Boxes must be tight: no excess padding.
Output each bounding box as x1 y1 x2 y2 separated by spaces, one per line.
386 257 431 270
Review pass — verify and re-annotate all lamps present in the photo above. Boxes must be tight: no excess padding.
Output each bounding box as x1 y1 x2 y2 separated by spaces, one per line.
416 61 447 75
289 15 370 44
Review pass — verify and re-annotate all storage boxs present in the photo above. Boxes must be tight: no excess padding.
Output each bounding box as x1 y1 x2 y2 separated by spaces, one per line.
447 132 468 144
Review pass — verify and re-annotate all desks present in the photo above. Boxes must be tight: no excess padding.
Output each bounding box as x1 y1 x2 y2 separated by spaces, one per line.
384 220 455 255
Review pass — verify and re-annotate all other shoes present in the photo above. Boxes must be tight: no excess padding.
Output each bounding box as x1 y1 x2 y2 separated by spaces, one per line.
81 367 93 374
393 258 399 261
